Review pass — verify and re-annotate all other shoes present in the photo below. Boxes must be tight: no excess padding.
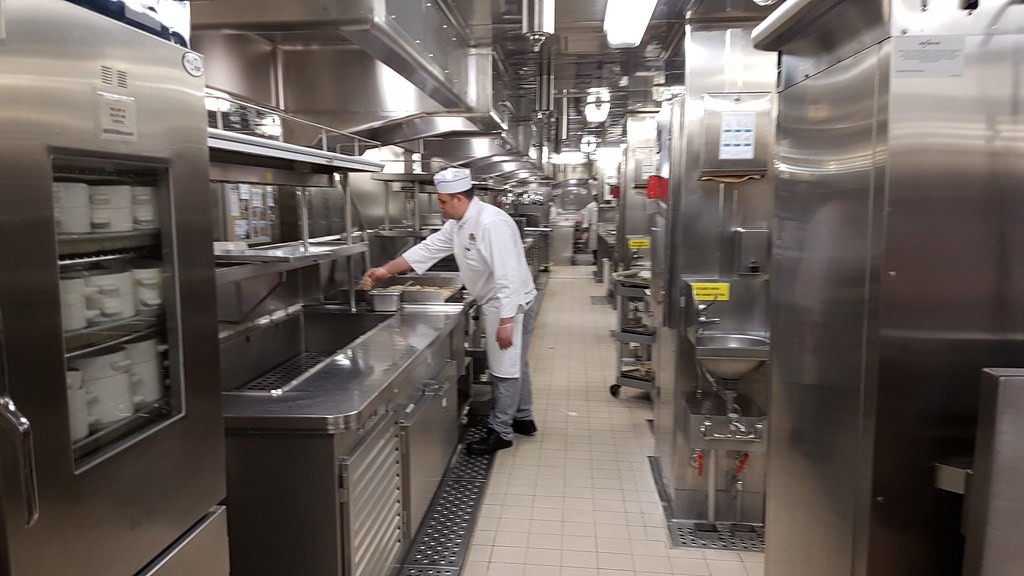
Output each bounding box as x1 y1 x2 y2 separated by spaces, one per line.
465 434 512 455
512 419 537 435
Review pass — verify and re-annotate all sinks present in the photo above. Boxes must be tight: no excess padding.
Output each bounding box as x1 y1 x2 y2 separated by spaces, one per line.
685 331 772 381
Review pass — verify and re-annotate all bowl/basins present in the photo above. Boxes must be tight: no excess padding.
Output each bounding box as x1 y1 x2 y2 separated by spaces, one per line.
369 289 403 310
421 213 442 225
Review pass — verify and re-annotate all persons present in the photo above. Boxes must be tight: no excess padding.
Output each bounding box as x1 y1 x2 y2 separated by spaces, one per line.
363 168 538 455
580 189 599 266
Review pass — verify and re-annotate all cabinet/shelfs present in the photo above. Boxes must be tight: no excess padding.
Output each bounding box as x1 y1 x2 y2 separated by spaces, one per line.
0 0 236 575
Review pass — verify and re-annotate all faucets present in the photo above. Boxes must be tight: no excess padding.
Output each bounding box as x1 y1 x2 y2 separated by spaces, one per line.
693 302 722 335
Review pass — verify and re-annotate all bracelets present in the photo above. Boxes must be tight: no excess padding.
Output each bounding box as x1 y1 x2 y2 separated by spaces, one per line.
497 323 513 328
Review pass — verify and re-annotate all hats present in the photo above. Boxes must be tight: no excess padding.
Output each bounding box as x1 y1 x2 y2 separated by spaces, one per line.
433 167 473 194
